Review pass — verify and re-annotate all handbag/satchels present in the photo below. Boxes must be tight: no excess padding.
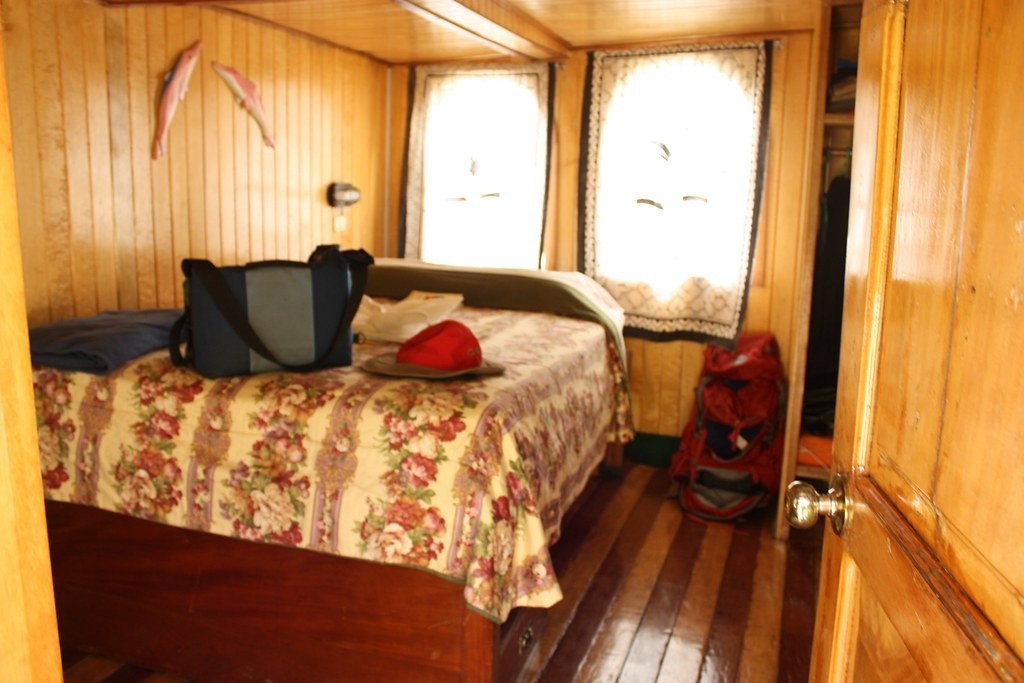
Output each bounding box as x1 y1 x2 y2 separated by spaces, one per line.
184 244 375 380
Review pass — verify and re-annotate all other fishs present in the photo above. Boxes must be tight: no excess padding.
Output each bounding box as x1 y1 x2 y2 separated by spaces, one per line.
146 40 207 163
209 57 277 150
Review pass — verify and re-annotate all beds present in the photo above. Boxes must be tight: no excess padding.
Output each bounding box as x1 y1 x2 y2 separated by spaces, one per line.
31 256 644 683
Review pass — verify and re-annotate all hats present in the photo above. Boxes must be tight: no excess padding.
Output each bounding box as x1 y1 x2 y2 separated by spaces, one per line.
361 319 508 380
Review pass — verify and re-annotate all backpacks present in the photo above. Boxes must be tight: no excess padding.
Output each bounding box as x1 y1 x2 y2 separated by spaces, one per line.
670 329 786 535
